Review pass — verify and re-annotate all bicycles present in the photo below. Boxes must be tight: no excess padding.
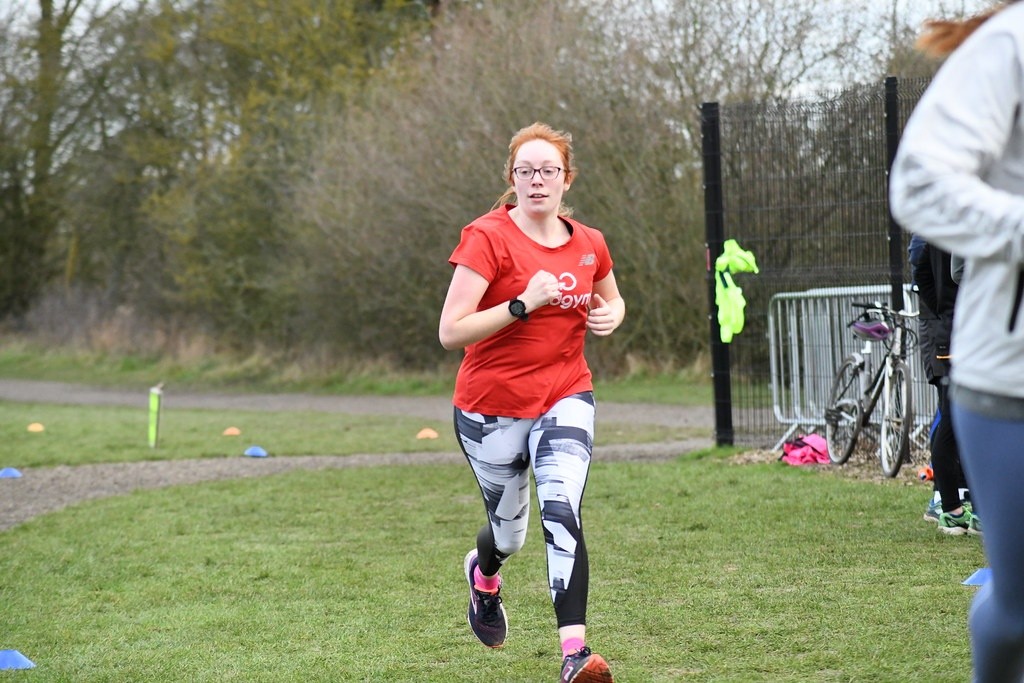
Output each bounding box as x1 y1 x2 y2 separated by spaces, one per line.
824 301 922 479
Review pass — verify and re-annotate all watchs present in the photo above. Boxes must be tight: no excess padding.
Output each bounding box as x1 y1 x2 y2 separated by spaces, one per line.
508 296 528 321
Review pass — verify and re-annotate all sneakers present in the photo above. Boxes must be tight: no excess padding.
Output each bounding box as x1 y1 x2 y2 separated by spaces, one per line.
965 515 986 537
559 647 614 683
924 498 946 523
463 547 509 650
939 506 973 536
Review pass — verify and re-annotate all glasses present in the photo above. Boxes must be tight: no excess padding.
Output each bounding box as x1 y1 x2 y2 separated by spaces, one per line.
512 166 566 181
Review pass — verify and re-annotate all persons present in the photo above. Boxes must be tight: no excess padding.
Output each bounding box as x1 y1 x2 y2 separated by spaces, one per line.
435 120 626 683
906 232 985 538
884 0 1023 683
